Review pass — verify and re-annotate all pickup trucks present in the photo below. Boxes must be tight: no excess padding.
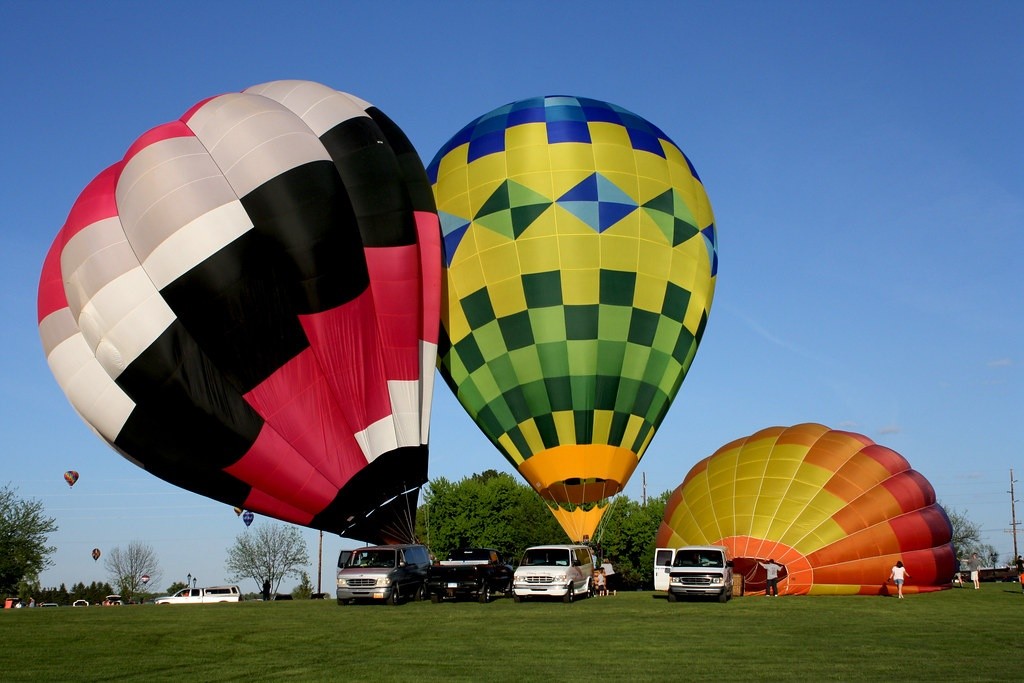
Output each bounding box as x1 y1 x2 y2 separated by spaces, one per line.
153 586 241 604
653 546 735 602
425 549 516 604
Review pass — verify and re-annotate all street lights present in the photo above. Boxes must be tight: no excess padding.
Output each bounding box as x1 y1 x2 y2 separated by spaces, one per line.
192 577 197 588
187 572 192 588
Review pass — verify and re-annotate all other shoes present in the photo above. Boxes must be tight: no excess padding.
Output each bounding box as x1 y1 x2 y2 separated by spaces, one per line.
775 595 778 597
899 595 905 599
766 594 770 597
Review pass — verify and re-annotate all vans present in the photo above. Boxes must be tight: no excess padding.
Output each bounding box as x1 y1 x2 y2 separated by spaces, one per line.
516 545 597 603
336 545 432 605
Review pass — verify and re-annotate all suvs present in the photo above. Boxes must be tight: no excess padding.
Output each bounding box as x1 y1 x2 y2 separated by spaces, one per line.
104 595 125 606
41 603 59 608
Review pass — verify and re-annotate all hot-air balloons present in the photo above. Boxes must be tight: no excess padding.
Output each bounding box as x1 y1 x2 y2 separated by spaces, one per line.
141 574 150 585
426 94 720 549
35 82 449 548
91 548 101 562
243 511 254 529
654 421 961 595
63 470 79 490
234 507 244 518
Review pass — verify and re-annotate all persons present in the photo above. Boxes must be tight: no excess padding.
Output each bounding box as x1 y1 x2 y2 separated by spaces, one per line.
181 591 189 596
429 555 443 565
887 561 911 599
1015 555 1024 590
262 580 271 601
962 553 979 590
13 598 26 608
141 596 145 604
601 557 617 596
758 559 785 596
102 598 118 606
26 596 35 607
953 559 963 588
596 567 605 596
590 550 598 569
